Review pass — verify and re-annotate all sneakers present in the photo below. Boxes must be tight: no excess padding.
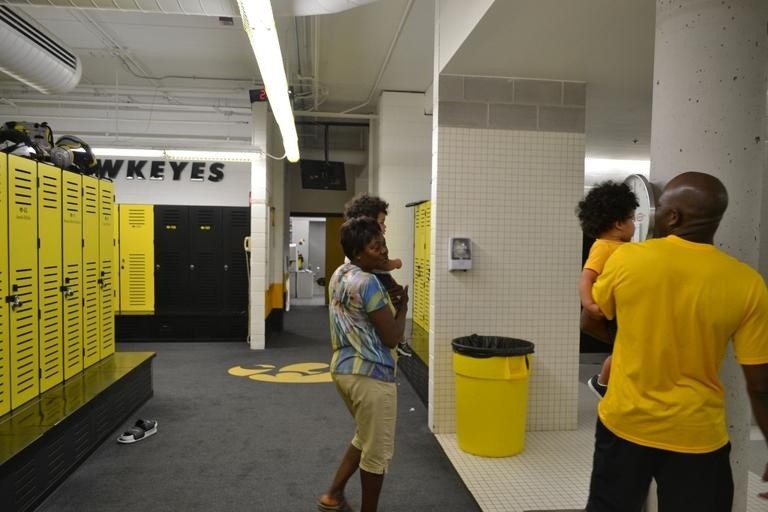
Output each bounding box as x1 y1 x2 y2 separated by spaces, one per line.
586 374 607 399
397 342 410 356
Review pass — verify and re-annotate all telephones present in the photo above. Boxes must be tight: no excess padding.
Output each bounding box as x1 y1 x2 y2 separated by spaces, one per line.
243 236 250 252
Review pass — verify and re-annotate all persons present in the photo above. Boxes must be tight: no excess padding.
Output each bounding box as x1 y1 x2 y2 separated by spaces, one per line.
582 171 768 512
574 179 640 401
315 215 410 512
343 193 413 358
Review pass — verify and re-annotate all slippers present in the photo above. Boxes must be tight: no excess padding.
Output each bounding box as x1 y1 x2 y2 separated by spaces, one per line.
318 498 354 512
135 419 157 428
116 424 158 444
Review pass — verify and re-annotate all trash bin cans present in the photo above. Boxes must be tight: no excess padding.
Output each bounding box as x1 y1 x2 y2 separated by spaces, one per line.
451 335 535 457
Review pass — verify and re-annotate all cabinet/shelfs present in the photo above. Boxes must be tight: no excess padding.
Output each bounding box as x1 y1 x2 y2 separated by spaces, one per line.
1 150 116 419
118 202 250 344
393 198 431 404
